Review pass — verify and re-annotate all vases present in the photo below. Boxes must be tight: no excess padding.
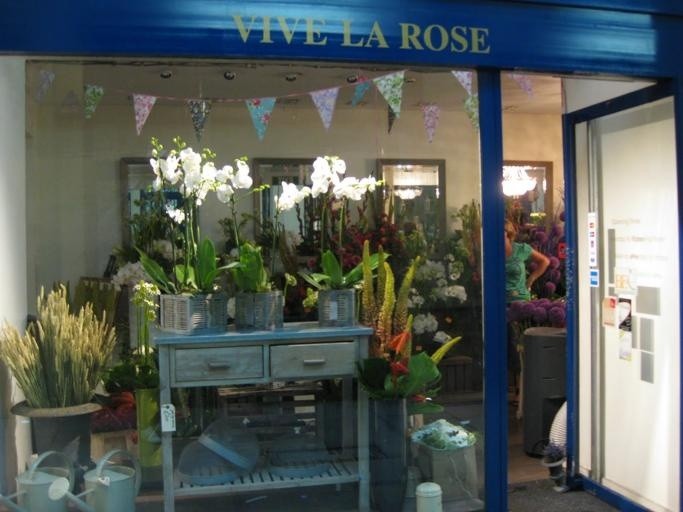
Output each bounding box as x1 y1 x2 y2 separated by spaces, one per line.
134 389 164 466
236 292 285 331
379 398 412 510
160 294 226 335
317 290 366 326
11 401 101 470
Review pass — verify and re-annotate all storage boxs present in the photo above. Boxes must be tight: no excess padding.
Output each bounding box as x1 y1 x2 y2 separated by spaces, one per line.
416 424 476 501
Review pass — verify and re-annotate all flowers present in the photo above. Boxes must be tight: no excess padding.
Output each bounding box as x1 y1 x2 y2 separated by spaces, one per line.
0 284 116 405
110 263 153 289
405 254 468 346
325 189 405 273
209 157 309 290
509 196 567 293
299 154 388 288
509 297 567 328
144 136 214 291
129 280 161 385
367 243 461 399
468 219 481 287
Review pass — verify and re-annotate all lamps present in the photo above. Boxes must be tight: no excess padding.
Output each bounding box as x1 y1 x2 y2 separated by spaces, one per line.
503 165 540 197
395 186 422 200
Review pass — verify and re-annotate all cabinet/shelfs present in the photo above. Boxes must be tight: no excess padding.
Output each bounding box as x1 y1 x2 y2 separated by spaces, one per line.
151 321 376 511
523 329 566 457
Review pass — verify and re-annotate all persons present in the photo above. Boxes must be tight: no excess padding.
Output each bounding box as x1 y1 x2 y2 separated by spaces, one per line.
500 217 551 404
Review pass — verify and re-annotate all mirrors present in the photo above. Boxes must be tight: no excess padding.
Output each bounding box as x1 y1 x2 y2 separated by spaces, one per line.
502 160 554 234
376 158 446 239
